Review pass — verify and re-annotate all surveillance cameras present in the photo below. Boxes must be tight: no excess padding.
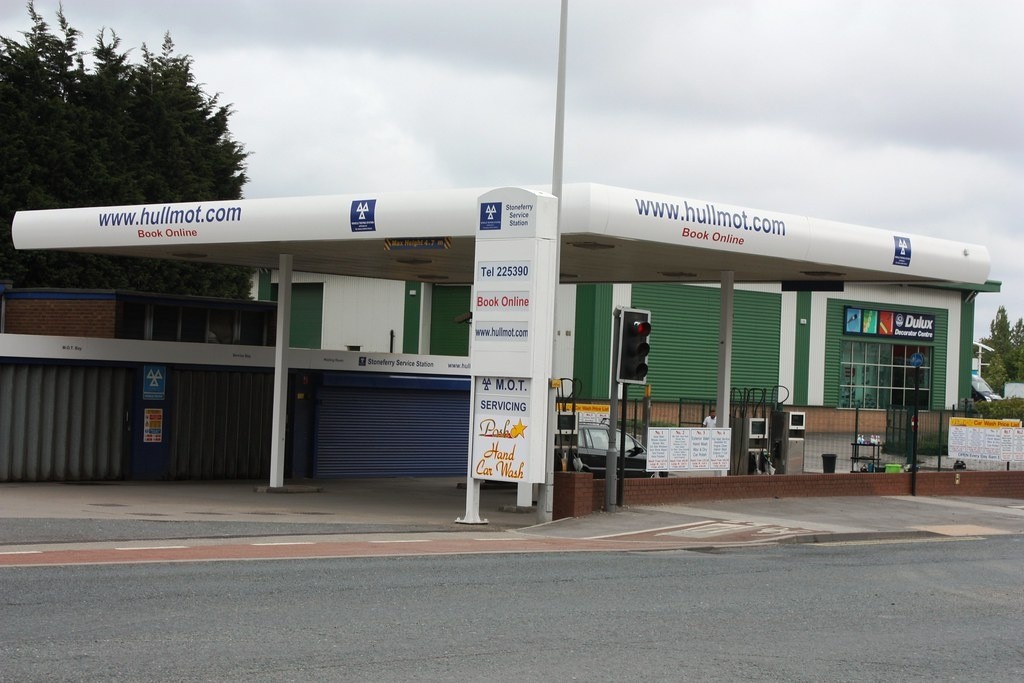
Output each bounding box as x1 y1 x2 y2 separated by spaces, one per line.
454 313 472 324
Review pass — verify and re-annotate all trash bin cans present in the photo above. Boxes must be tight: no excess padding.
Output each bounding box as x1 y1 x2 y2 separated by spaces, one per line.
821 453 837 475
953 460 966 471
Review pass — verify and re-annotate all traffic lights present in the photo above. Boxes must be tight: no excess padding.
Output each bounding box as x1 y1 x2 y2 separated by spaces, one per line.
616 307 653 385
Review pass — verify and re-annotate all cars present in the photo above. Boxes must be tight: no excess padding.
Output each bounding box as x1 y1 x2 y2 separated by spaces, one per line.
577 420 668 478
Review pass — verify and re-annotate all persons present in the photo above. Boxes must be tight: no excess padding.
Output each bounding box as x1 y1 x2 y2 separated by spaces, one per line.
702 409 718 428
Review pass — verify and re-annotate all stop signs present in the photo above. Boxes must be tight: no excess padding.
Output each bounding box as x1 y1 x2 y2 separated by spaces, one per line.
911 416 919 431
